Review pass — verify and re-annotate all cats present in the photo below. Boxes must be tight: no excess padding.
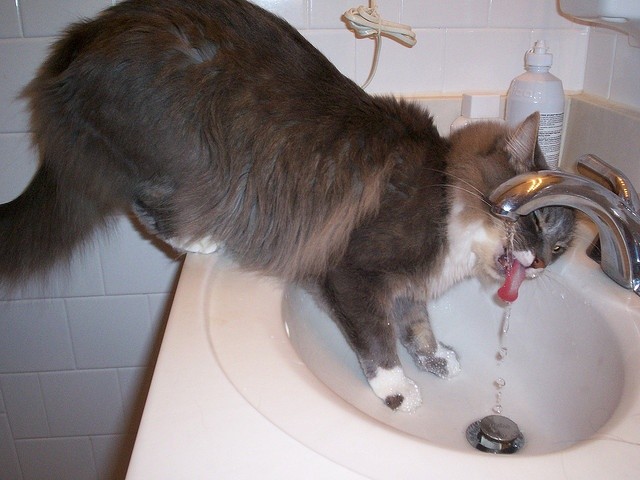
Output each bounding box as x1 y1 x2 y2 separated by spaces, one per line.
1 0 582 416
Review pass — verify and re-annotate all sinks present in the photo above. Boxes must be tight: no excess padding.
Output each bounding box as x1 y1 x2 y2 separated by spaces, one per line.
127 220 640 480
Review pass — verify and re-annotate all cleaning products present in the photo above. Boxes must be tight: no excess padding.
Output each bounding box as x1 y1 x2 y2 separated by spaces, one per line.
504 40 564 170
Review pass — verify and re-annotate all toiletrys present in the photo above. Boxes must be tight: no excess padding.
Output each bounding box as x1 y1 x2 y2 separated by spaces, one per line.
448 91 504 136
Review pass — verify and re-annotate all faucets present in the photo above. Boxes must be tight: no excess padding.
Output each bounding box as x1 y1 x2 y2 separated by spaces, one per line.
488 170 640 296
